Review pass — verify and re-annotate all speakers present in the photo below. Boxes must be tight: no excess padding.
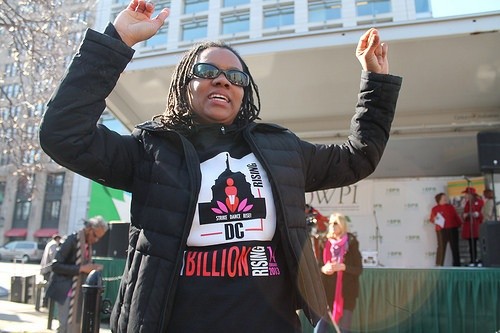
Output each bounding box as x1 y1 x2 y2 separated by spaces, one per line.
479 222 500 268
477 131 500 173
107 222 130 258
92 229 110 257
10 275 35 303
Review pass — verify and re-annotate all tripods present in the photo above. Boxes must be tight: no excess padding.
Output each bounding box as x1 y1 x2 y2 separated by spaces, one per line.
374 227 384 267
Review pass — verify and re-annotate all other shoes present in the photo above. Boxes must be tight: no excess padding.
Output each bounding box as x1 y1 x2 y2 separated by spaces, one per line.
468 260 482 267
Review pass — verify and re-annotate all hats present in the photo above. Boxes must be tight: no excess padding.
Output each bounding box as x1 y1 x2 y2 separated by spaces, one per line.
461 186 478 194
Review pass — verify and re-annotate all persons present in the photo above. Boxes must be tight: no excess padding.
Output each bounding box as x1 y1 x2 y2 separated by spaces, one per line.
40 234 61 313
44 216 108 333
430 193 463 267
314 212 363 333
479 189 494 221
39 0 404 333
461 185 485 268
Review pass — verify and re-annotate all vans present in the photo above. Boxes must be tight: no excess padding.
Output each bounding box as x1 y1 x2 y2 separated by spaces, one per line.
0 240 45 263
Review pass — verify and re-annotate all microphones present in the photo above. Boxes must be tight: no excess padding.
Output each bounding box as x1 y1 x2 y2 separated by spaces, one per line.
461 174 471 183
373 210 376 215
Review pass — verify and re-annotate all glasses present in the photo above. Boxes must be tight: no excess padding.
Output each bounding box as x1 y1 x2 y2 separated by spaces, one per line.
185 62 250 87
